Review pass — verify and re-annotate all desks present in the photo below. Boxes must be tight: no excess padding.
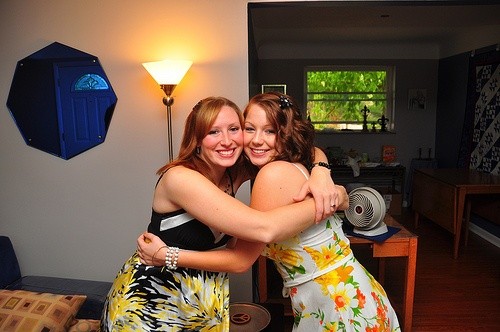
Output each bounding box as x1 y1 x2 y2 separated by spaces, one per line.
407 159 500 258
340 214 419 332
331 160 407 216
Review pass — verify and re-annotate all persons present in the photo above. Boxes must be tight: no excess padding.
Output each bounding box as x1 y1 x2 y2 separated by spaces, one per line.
101 97 350 332
136 92 403 332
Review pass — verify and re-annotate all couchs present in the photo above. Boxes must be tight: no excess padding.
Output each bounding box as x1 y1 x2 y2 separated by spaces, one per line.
0 235 113 318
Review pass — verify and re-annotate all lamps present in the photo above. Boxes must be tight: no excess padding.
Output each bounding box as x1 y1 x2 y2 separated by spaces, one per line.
143 61 194 163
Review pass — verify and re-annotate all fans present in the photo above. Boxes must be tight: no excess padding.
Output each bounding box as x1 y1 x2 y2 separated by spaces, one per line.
344 187 389 237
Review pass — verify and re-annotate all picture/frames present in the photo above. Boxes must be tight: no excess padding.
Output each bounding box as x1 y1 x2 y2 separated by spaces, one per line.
262 84 287 96
408 88 426 112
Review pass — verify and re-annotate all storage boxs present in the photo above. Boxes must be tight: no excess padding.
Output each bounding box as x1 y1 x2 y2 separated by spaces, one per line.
373 188 402 216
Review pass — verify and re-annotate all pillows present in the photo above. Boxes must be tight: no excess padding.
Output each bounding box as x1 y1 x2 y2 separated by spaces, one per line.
68 319 101 332
0 289 88 332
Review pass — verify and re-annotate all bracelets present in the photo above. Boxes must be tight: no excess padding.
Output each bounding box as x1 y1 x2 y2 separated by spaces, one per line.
152 246 169 268
166 246 179 270
311 162 332 170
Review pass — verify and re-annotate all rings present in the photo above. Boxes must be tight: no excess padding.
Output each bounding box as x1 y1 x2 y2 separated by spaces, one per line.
330 204 336 208
